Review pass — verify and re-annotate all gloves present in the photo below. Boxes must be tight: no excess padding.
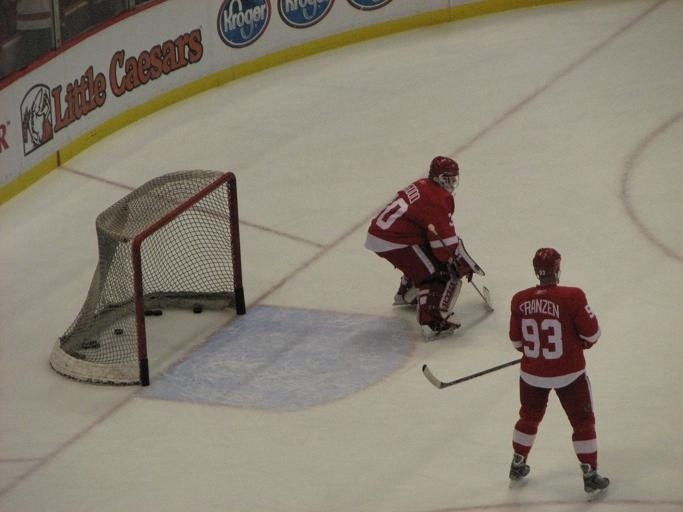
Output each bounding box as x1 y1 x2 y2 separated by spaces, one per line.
447 255 473 284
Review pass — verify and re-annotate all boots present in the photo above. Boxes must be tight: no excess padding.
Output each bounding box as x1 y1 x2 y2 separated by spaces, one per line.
507 452 530 481
416 302 458 332
579 462 609 492
393 278 417 304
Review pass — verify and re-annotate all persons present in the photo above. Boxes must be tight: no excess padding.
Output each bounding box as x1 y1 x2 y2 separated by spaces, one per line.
6 0 67 72
506 247 609 493
362 154 487 332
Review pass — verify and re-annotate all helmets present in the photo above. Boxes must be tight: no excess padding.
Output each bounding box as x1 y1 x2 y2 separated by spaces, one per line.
532 247 561 276
428 155 459 178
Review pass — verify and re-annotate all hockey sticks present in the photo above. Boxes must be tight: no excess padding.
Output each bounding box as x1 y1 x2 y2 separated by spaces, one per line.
428 224 492 311
422 359 521 388
